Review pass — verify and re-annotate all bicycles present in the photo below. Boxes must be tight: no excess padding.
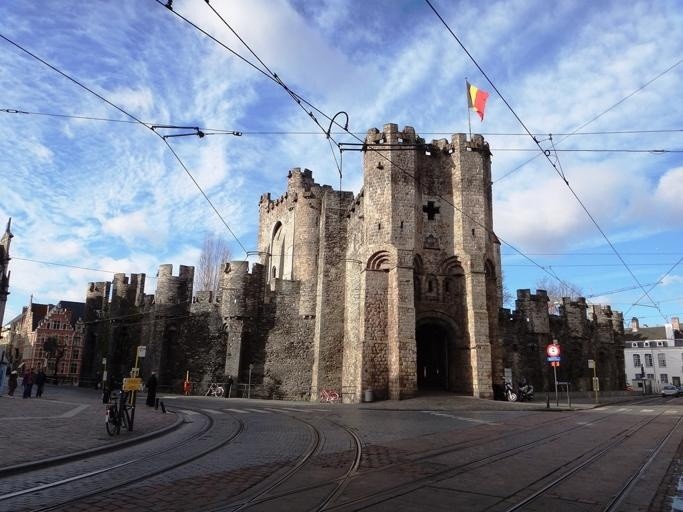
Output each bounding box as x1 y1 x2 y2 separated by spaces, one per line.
105 389 133 435
316 384 342 405
201 381 224 400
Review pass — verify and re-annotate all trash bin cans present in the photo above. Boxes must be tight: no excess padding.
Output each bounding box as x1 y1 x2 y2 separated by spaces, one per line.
364 390 374 403
229 385 237 397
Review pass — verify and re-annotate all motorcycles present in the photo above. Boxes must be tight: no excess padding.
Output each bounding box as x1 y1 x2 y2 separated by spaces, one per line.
494 378 536 403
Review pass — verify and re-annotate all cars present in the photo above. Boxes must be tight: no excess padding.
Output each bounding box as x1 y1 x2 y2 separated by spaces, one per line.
660 383 683 397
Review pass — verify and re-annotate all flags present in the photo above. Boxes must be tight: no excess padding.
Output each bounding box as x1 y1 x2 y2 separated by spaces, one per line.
467 82 490 122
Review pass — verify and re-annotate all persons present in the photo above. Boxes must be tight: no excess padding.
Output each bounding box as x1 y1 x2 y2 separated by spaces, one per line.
146 373 158 407
224 375 233 398
8 371 17 399
34 369 50 398
21 368 34 398
